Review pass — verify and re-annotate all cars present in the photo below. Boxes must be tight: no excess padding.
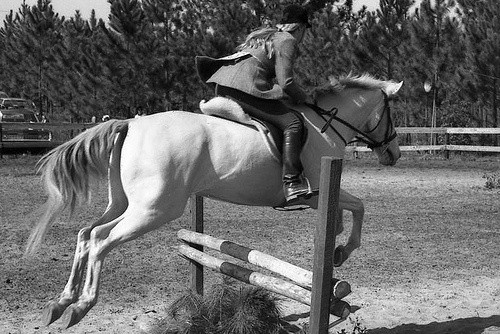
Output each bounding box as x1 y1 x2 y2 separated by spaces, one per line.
0 98 51 155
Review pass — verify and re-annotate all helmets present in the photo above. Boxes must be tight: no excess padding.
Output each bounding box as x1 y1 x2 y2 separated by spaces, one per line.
280 5 312 29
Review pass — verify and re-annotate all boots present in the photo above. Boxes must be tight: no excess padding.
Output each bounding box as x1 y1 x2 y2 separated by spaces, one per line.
283 132 320 201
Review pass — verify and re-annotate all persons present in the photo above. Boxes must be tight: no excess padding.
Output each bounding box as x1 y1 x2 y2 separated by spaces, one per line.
194 3 318 201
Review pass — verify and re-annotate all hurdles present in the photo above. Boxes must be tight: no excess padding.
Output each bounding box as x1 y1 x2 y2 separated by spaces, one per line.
176 156 365 334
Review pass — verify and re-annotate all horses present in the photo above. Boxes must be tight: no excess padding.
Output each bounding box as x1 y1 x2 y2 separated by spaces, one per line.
23 69 405 330
